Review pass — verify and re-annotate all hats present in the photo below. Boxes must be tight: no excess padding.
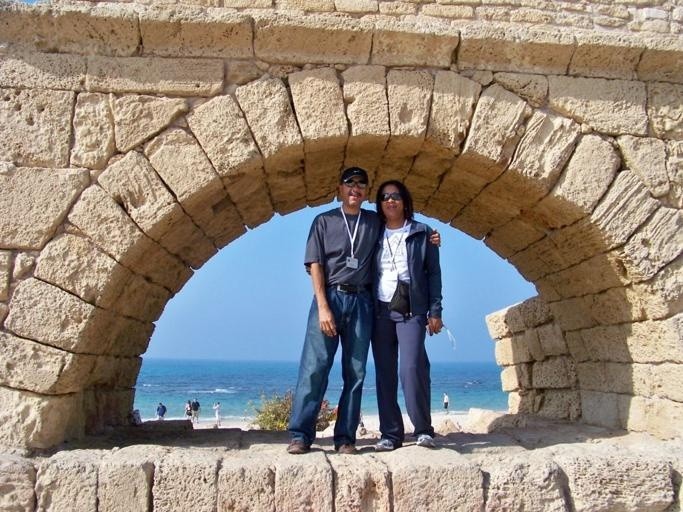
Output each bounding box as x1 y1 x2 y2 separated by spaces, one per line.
342 167 368 183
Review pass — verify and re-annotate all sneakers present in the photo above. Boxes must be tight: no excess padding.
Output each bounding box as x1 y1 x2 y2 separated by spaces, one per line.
288 441 310 454
375 438 394 451
418 434 436 447
339 444 355 453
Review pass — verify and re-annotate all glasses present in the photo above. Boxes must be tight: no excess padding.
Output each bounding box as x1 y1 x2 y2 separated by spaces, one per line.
382 193 402 201
345 180 367 188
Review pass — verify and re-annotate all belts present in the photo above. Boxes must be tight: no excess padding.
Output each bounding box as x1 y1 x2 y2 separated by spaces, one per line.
334 284 368 293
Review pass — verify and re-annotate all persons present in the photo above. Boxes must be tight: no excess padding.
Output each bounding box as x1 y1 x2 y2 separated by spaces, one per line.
155 402 166 419
184 400 192 420
443 391 450 414
212 401 221 426
284 165 440 455
191 398 201 423
370 180 443 452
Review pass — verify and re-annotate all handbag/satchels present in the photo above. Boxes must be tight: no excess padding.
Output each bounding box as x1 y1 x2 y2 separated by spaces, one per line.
388 280 411 315
